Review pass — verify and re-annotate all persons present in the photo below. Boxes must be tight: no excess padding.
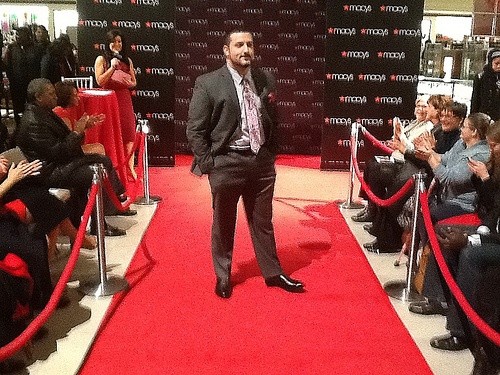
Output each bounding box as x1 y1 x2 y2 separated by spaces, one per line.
0 23 139 375
187 29 305 298
470 48 500 121
351 94 500 374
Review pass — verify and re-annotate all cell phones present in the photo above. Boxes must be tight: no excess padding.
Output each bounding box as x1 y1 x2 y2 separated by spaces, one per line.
465 156 472 163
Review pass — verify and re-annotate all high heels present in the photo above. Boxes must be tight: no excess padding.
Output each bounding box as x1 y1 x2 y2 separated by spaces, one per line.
69 237 98 250
127 168 140 182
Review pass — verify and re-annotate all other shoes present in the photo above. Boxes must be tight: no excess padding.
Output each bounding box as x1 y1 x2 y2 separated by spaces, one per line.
33 328 48 343
103 208 138 216
33 293 70 309
119 194 128 202
90 222 127 236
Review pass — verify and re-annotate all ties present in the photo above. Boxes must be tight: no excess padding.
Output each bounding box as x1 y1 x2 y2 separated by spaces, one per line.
241 80 263 155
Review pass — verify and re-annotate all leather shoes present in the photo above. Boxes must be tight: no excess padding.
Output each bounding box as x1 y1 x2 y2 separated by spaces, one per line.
363 224 379 237
351 208 372 222
409 301 442 314
265 274 303 292
363 240 399 252
430 335 468 351
215 279 231 298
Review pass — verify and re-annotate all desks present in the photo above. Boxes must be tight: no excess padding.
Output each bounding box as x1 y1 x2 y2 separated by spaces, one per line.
77 86 129 212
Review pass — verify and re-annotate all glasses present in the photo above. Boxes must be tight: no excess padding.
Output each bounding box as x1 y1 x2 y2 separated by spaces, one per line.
461 125 470 130
439 114 457 119
